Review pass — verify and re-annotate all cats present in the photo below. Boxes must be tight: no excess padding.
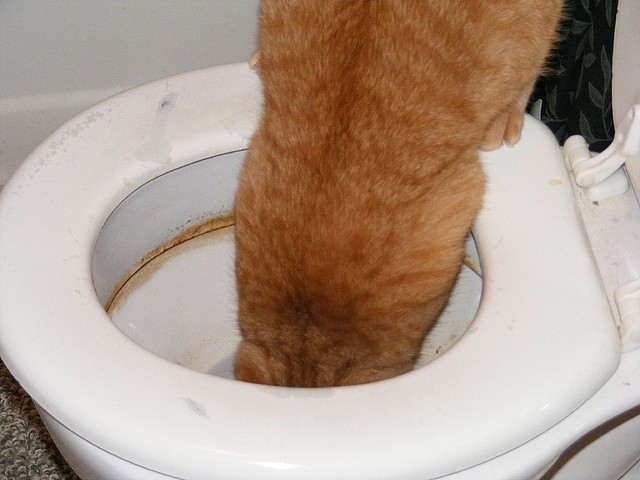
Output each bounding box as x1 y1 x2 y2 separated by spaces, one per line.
233 0 565 388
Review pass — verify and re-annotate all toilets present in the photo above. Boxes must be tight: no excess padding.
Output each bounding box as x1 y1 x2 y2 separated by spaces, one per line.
0 0 640 479
